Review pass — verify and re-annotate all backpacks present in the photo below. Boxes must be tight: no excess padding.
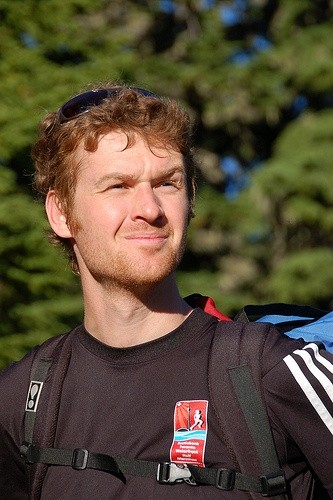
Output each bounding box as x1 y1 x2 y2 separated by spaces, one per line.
234 302 333 353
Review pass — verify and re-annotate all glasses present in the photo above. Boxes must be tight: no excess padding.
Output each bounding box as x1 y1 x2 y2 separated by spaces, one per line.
44 87 159 134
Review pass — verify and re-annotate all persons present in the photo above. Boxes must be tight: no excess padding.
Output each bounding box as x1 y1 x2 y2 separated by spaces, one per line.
1 79 332 499
